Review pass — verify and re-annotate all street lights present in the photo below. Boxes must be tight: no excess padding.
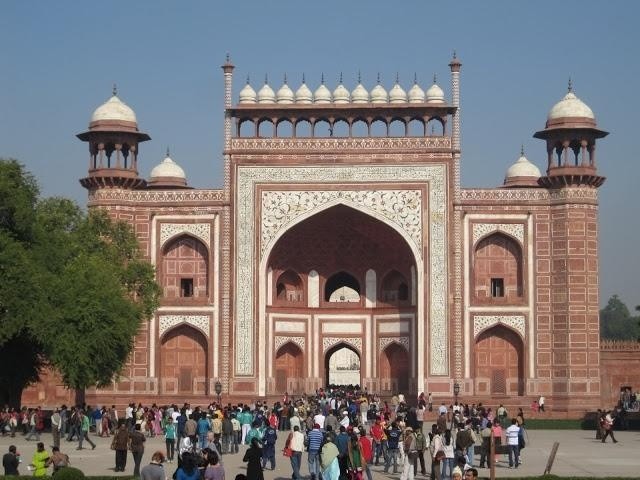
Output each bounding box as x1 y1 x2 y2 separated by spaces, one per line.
454 380 459 402
215 377 221 403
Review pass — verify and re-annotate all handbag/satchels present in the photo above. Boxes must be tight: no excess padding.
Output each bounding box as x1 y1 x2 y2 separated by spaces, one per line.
111 429 118 450
435 451 446 461
408 450 420 459
283 433 293 457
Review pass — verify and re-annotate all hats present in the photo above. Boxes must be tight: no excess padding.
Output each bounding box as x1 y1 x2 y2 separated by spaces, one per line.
404 427 413 432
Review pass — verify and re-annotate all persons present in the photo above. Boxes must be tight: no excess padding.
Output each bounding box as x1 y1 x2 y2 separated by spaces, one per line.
532 395 544 413
0 385 526 480
620 387 640 410
595 408 618 444
